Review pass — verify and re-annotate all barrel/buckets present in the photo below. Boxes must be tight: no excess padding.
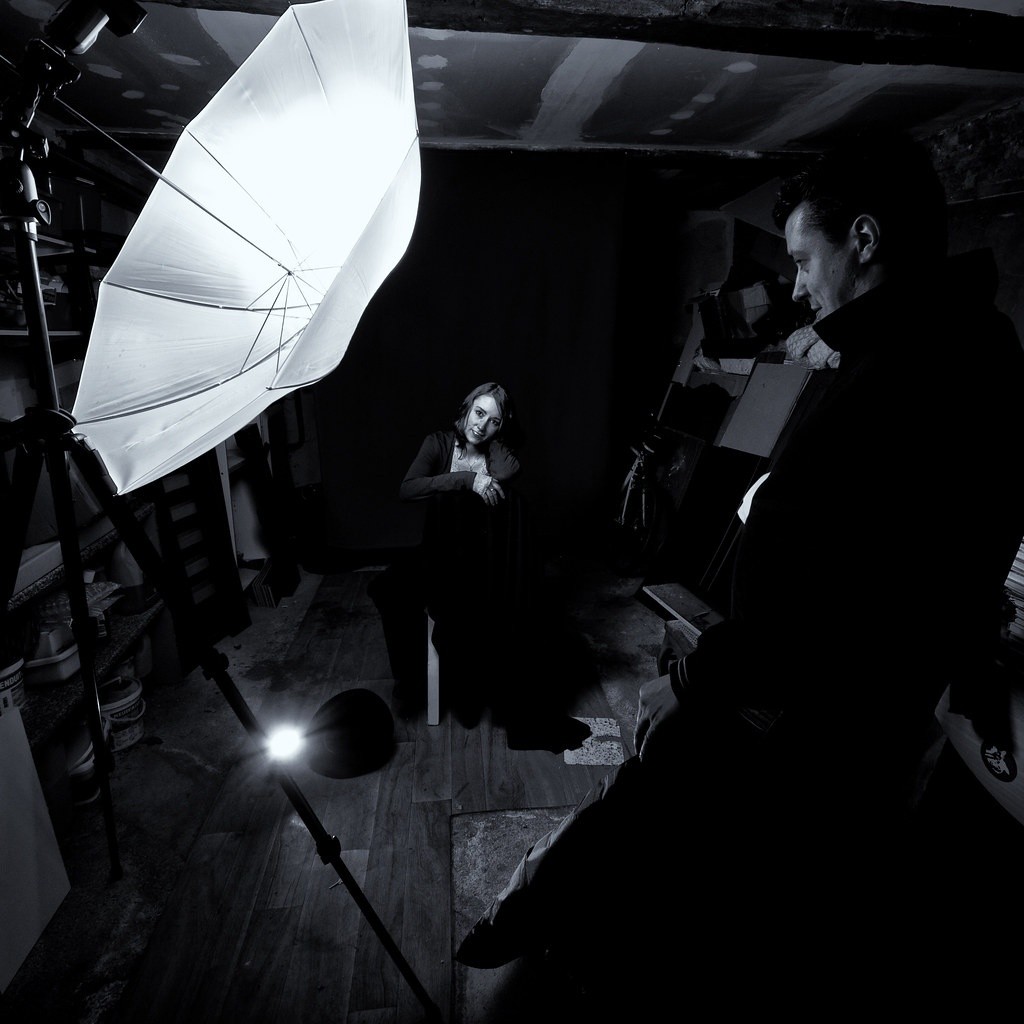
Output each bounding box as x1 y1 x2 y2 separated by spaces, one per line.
99 675 148 752
117 654 137 681
60 730 100 806
0 656 28 713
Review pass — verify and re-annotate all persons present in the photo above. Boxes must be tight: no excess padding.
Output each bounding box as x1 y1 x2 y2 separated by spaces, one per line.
628 167 1024 984
371 383 545 752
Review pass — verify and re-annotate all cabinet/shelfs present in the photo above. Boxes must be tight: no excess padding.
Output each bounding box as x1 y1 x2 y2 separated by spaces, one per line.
0 56 279 758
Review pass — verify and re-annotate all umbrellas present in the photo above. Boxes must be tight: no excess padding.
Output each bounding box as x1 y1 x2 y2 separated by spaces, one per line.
71 1 423 501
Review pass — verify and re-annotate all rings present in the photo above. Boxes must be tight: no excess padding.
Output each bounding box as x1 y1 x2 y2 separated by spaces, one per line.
490 488 494 491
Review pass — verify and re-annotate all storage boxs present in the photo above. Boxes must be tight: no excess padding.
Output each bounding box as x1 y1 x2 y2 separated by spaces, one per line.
24 645 80 681
